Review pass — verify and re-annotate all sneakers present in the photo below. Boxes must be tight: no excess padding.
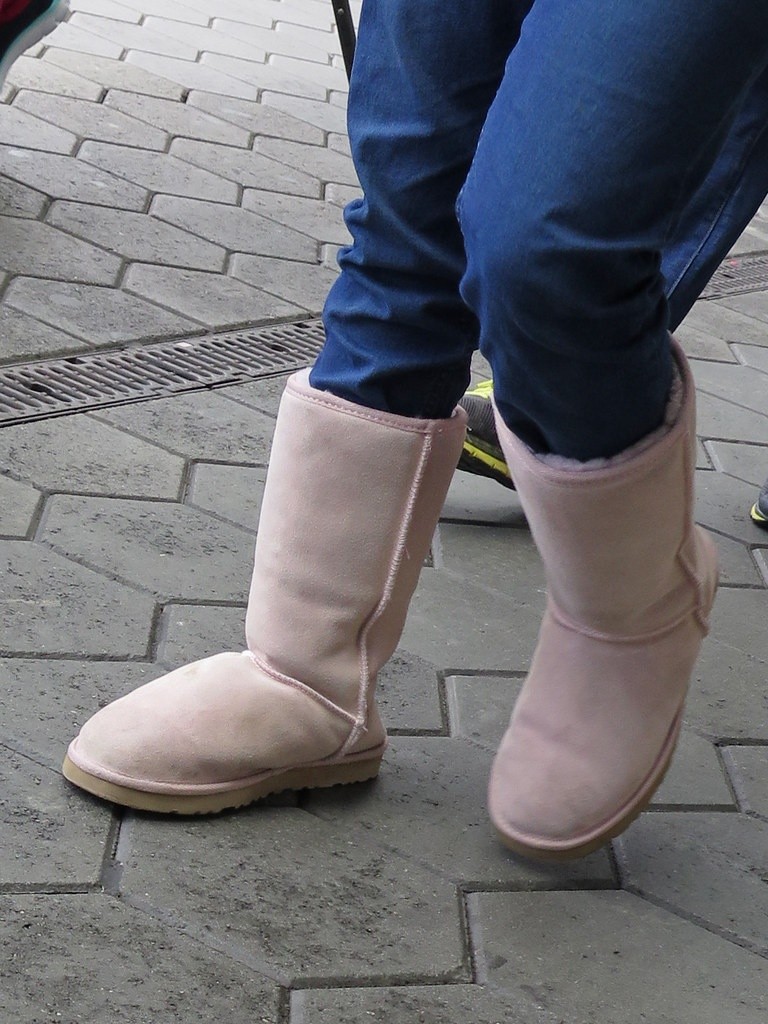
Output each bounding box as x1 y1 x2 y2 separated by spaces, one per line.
437 384 525 528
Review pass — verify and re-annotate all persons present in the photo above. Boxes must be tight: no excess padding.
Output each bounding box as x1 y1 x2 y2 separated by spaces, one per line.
61 0 768 856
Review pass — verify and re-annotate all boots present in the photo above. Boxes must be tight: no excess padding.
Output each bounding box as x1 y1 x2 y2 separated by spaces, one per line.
480 334 721 856
56 366 472 818
